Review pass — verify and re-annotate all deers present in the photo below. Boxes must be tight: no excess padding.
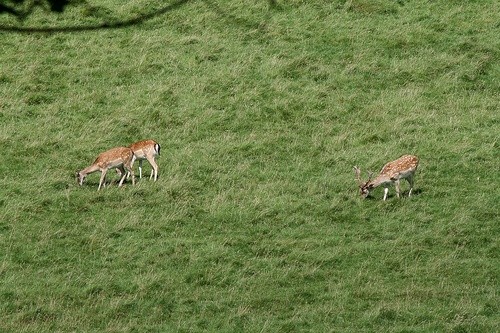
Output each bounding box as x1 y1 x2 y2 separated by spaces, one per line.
115 139 161 184
353 153 420 203
74 146 136 194
